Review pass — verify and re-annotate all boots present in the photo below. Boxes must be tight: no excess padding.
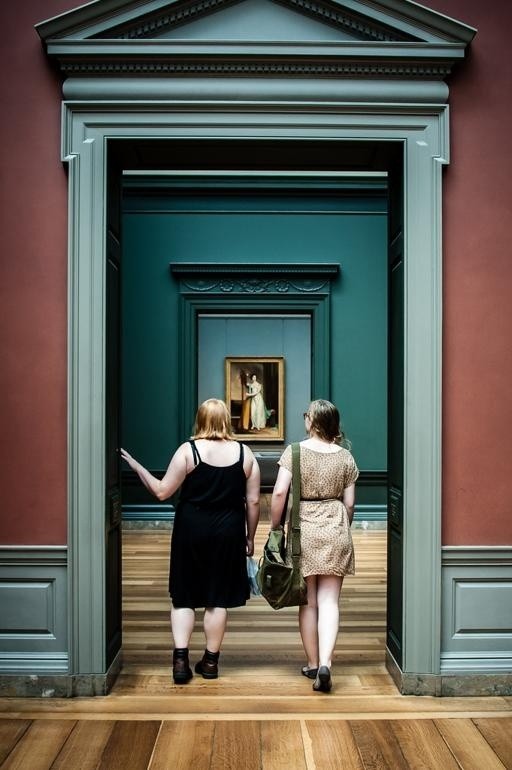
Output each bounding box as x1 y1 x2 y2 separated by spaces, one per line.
173 649 193 684
195 648 220 679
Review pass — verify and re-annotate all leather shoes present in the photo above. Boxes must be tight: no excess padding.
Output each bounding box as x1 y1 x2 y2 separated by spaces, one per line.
311 666 332 691
301 664 318 680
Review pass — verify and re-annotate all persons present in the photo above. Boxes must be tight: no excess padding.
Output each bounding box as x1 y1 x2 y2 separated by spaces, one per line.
270 398 362 691
244 373 271 431
120 397 263 683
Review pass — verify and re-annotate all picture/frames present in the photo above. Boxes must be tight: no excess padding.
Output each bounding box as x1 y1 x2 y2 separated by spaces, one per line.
225 356 285 443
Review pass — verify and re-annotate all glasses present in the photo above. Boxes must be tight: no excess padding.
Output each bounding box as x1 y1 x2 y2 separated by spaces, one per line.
303 413 309 420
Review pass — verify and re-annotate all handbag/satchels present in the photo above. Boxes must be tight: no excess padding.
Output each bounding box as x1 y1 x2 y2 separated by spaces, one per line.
255 527 304 608
